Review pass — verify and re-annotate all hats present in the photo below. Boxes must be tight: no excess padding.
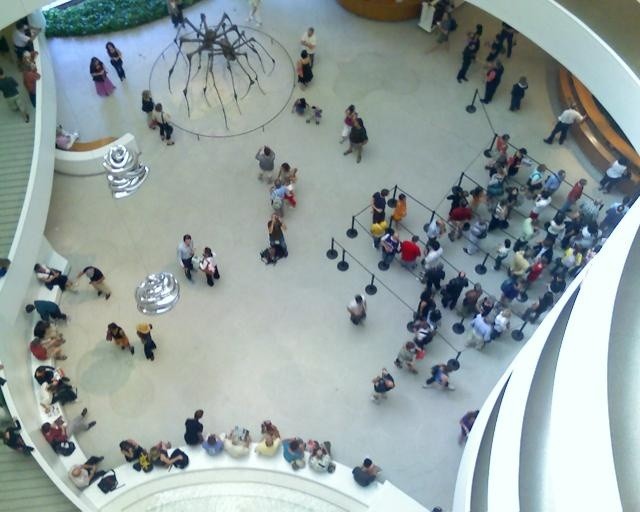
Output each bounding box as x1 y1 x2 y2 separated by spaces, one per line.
134 321 151 334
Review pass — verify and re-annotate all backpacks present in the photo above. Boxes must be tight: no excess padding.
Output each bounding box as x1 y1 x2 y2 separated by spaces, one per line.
423 333 433 344
54 441 75 455
98 475 118 493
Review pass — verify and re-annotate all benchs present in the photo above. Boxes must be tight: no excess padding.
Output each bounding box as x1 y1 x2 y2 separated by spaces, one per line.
56 132 118 151
26 252 98 493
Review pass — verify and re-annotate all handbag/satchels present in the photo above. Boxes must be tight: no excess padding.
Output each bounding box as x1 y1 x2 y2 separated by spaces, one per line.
430 364 440 376
200 265 215 275
33 321 49 338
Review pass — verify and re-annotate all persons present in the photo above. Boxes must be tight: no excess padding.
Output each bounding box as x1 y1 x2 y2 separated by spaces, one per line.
292 27 324 127
340 103 359 144
139 88 175 147
348 294 366 323
435 1 528 110
246 1 263 26
352 458 376 487
344 118 368 163
542 102 587 144
256 146 275 186
0 18 43 121
167 0 185 30
106 41 128 85
88 57 115 97
